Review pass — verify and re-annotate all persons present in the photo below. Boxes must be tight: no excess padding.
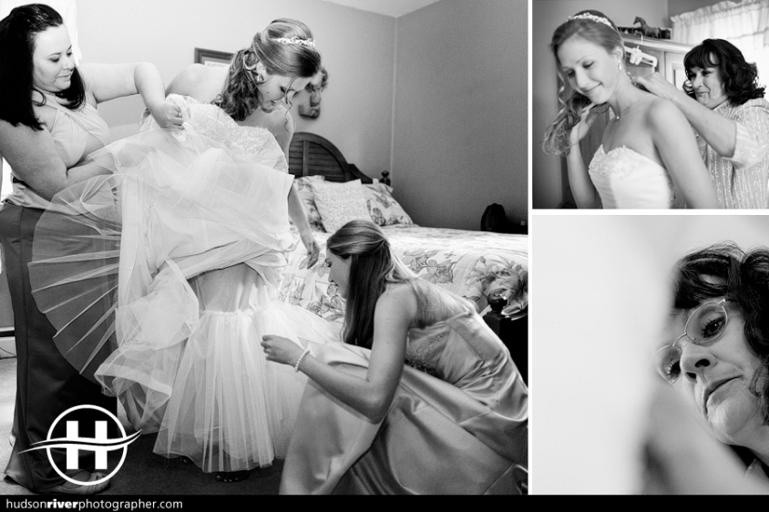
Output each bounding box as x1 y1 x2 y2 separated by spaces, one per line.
631 37 769 210
23 17 342 486
539 8 720 210
261 219 528 497
0 0 186 498
654 238 769 491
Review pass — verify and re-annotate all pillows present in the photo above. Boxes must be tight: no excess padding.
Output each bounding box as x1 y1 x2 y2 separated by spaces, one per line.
287 174 326 232
306 176 374 233
359 183 415 226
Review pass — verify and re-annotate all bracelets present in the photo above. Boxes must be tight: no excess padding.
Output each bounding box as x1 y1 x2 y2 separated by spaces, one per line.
294 348 310 373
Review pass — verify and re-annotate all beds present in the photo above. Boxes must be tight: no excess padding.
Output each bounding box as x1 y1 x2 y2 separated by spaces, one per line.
286 131 528 384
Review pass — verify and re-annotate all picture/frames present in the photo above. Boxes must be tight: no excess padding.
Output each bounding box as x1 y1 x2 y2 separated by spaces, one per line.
193 47 235 66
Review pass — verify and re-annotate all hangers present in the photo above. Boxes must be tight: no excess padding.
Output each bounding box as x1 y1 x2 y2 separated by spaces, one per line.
622 30 658 71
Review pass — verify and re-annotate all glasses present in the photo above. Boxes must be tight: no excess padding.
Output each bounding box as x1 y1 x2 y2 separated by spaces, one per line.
654 297 739 386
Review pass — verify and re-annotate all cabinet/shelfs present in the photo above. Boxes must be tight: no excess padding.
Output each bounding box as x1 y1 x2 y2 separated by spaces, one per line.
557 31 696 209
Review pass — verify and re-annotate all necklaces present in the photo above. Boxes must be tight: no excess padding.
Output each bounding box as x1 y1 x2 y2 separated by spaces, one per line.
614 93 652 120
